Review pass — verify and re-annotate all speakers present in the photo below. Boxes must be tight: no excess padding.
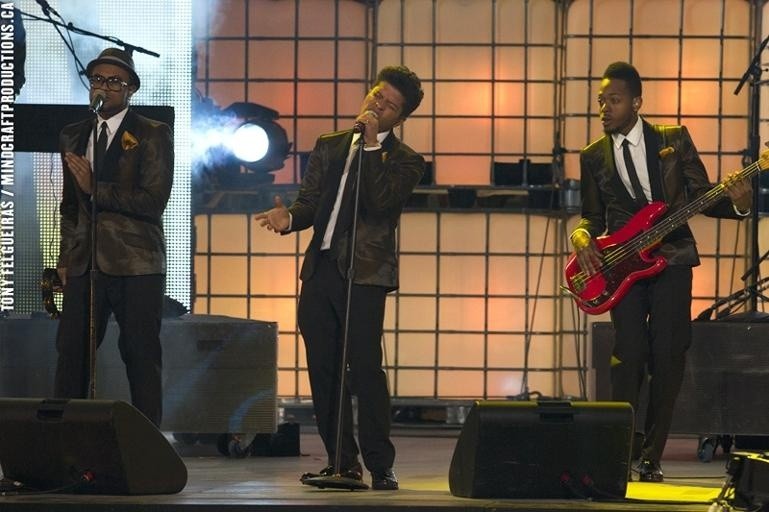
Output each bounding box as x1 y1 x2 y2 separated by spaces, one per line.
449 401 634 502
1 397 187 494
0 320 278 434
592 322 769 437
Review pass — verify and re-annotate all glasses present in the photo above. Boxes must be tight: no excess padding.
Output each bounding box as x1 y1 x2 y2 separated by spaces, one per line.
88 73 133 92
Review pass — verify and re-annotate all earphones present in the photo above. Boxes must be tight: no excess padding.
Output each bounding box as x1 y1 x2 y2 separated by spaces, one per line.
633 98 640 107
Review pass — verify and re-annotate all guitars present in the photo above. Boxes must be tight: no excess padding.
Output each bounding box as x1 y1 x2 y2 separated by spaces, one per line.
565 149 769 315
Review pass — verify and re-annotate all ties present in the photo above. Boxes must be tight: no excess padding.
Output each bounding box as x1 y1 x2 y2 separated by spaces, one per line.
94 122 108 167
622 138 649 210
328 145 361 258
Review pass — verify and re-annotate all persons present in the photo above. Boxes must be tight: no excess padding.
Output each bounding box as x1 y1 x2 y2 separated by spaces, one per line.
569 62 753 480
255 64 427 491
50 48 174 428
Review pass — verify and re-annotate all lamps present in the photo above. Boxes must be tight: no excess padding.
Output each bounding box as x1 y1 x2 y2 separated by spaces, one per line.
217 118 295 188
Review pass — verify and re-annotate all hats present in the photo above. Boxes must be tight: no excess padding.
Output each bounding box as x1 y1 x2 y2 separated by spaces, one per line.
85 48 140 94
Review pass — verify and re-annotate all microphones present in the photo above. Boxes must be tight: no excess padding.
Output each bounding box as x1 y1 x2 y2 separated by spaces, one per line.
88 90 107 113
353 110 378 134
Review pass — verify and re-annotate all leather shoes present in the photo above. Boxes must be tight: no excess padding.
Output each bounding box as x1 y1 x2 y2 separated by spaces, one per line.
301 463 364 480
367 465 400 490
638 458 665 482
